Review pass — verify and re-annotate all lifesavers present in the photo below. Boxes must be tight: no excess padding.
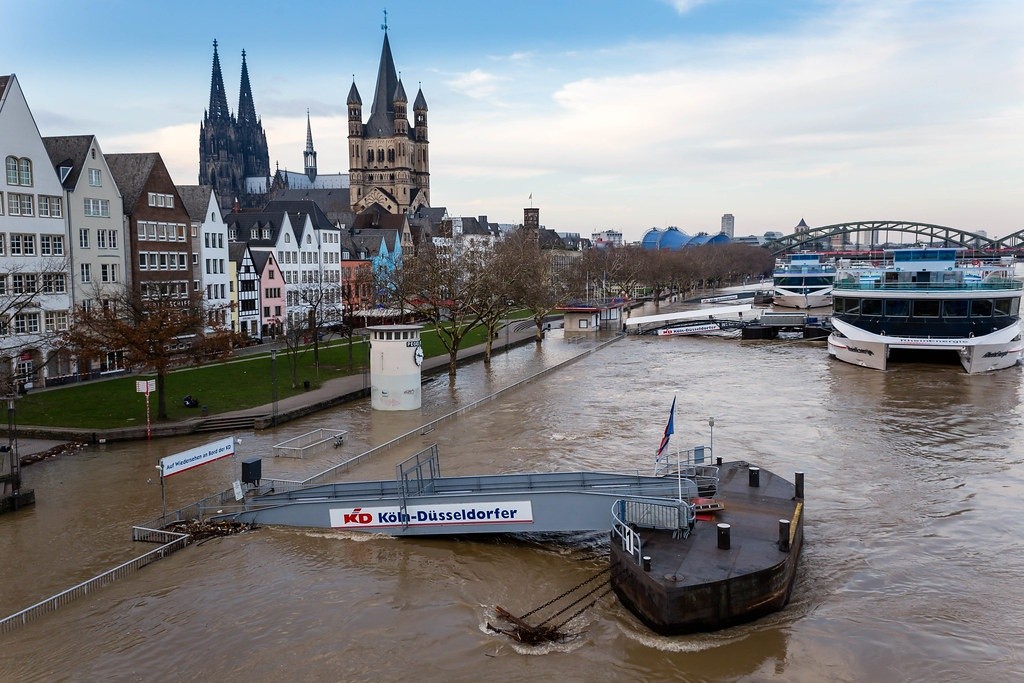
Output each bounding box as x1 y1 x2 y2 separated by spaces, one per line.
972 259 979 266
872 261 878 267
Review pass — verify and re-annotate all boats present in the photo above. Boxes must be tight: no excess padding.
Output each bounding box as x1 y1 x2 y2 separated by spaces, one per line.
610 418 806 638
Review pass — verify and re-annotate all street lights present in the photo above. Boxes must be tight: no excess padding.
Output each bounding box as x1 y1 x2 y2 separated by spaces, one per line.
271 349 277 414
6 393 16 466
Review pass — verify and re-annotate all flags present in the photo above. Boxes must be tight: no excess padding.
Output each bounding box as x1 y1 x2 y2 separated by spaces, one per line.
655 395 676 462
